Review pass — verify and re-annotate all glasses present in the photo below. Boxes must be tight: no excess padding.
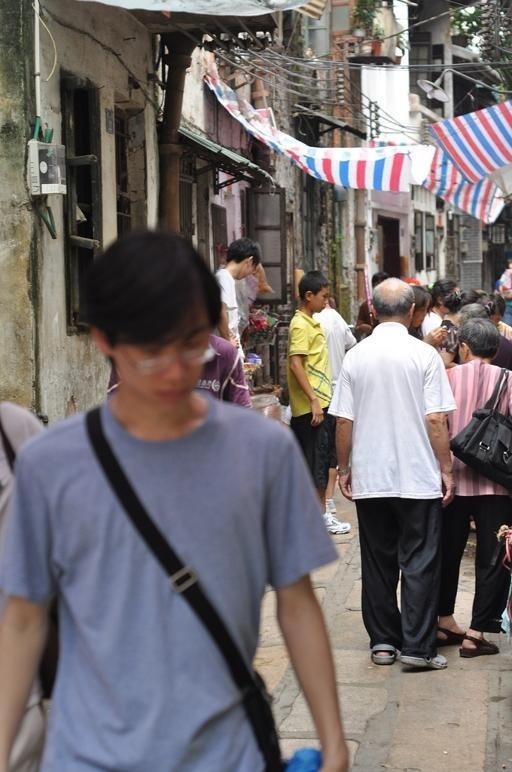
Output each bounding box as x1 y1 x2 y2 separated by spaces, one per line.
121 339 215 376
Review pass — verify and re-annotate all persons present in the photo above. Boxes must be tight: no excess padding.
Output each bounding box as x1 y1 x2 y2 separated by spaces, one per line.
326 279 448 670
407 287 448 347
421 279 511 529
313 301 357 514
107 335 252 407
1 401 49 770
353 272 387 341
286 270 347 535
2 230 348 770
214 238 261 356
445 319 512 657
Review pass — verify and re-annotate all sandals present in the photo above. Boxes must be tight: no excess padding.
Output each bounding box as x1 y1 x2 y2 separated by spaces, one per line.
372 624 498 669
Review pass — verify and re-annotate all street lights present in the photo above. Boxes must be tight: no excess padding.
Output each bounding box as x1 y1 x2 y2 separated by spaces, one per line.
409 58 512 111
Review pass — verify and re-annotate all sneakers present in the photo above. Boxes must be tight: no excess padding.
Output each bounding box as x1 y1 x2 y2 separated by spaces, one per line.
325 498 337 513
322 513 351 535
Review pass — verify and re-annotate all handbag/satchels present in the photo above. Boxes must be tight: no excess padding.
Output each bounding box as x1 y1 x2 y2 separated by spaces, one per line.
450 408 512 492
